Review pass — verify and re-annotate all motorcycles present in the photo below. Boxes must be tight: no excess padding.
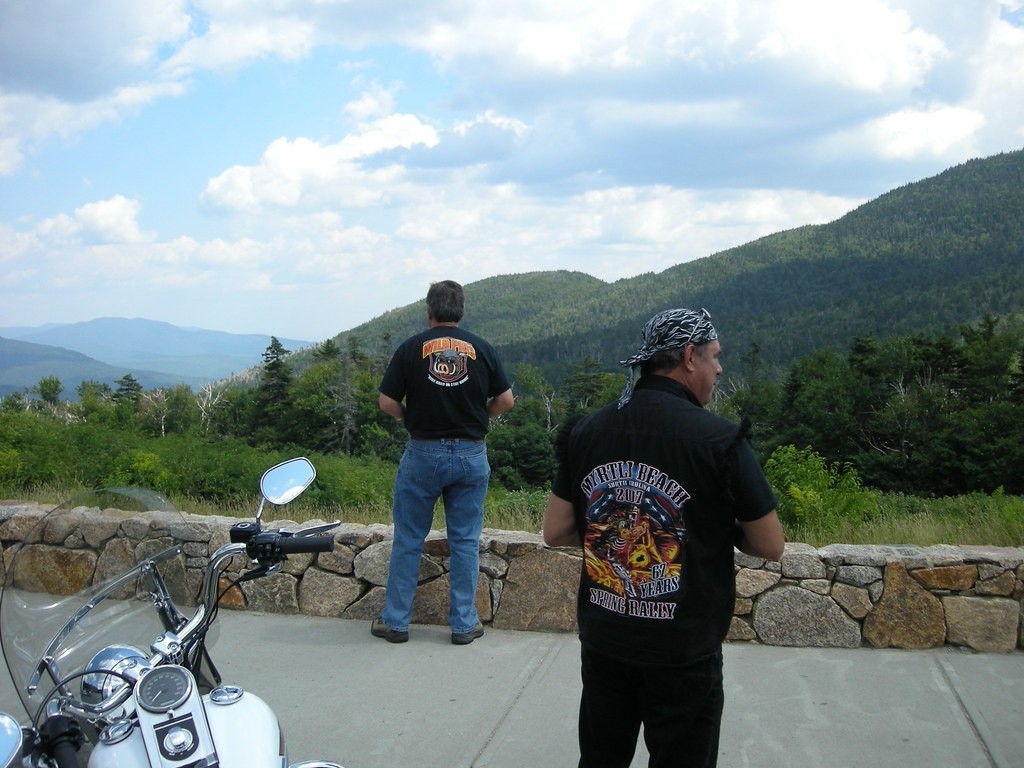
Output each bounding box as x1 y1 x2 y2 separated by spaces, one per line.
1 457 345 768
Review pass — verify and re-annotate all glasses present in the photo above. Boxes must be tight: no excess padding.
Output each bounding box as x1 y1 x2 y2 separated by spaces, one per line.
685 308 712 348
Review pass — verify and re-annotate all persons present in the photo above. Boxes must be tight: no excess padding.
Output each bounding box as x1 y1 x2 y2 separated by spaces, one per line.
544 308 784 768
370 280 514 645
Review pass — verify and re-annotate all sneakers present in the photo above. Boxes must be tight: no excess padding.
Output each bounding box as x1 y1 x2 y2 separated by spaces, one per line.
370 617 409 643
452 621 484 644
